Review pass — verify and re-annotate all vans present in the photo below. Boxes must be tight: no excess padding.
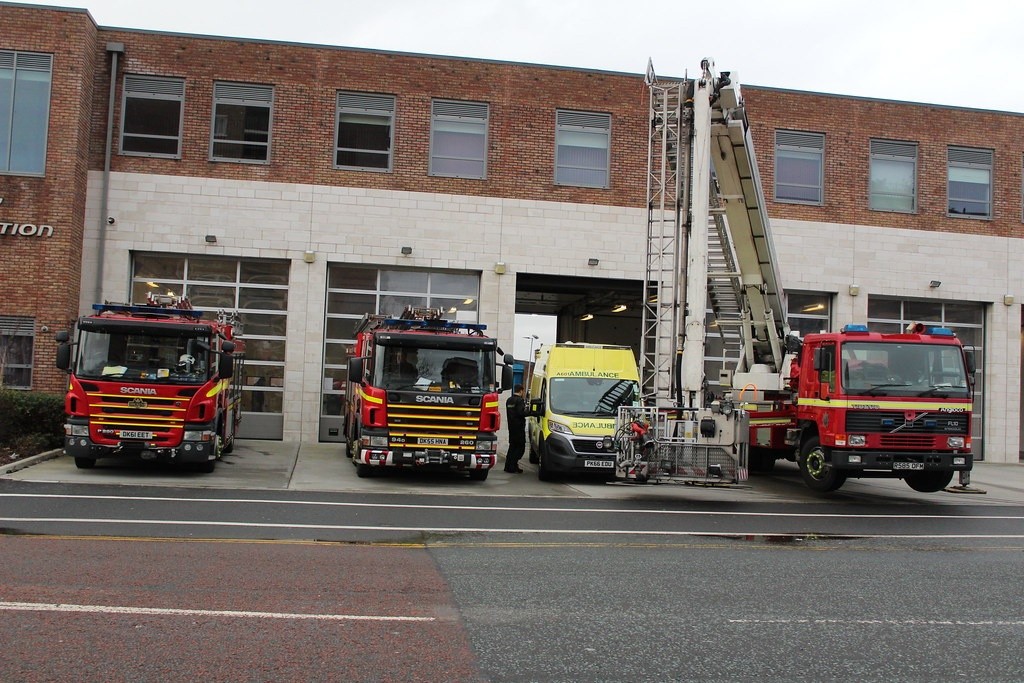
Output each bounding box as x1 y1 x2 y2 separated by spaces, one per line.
528 341 647 480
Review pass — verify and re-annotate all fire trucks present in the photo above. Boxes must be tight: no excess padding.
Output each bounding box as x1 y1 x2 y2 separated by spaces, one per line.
613 55 988 494
55 291 247 474
343 305 514 483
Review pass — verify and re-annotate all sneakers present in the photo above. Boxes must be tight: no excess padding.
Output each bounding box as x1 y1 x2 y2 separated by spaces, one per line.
504 465 523 474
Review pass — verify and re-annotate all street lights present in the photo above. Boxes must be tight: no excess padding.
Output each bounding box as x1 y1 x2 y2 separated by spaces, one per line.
521 334 539 401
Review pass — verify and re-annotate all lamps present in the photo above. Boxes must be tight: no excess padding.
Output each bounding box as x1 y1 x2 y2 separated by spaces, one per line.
580 313 593 321
611 304 627 312
401 247 412 257
930 281 941 287
802 303 825 312
588 259 599 265
205 235 217 243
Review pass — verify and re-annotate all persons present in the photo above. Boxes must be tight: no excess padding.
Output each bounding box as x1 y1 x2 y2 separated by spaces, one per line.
503 384 532 473
